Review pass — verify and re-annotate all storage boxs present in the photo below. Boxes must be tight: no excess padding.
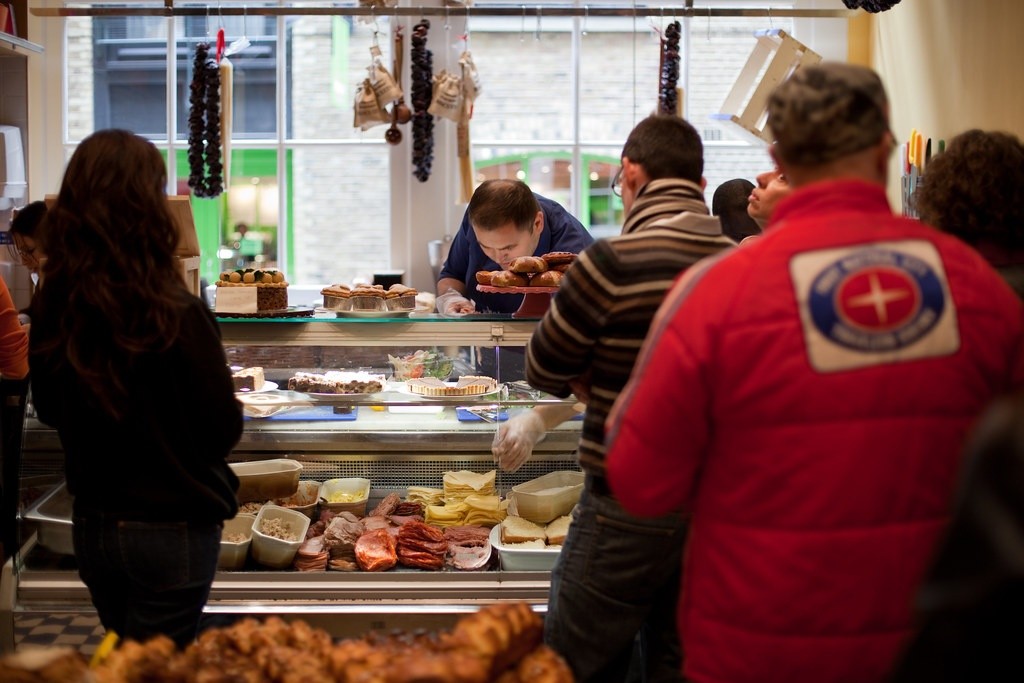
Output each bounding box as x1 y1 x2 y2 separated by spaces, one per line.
227 458 303 505
216 515 257 572
318 477 372 521
45 194 202 299
489 524 562 571
238 480 322 526
512 470 586 524
21 481 75 555
250 505 311 570
705 28 823 150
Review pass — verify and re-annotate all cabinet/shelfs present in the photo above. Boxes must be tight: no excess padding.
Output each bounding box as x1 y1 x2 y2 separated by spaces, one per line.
0 312 586 643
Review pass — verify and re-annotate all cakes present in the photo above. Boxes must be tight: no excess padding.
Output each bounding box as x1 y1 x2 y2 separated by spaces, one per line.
215 268 288 312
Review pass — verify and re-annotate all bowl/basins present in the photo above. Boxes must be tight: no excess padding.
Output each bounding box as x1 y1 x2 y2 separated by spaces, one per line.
251 504 311 570
488 523 561 571
279 480 319 519
228 459 303 504
512 471 585 523
220 516 255 568
319 477 370 516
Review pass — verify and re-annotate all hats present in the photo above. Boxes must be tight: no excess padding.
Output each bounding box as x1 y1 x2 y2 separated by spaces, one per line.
768 62 898 164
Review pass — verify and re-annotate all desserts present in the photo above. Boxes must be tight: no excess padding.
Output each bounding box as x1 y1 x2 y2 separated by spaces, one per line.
320 283 417 311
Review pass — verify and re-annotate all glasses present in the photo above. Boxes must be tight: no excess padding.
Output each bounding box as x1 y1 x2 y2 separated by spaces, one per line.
17 246 37 261
611 158 637 197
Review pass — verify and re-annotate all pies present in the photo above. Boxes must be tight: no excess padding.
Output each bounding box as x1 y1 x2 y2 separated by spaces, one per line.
404 375 497 395
288 371 386 394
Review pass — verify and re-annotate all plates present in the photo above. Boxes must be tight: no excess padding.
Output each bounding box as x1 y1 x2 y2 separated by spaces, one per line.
335 310 410 318
403 381 501 401
307 393 370 402
234 381 279 394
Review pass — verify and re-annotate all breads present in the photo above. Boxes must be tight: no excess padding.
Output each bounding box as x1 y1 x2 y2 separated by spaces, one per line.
230 366 265 391
475 251 580 287
502 514 573 548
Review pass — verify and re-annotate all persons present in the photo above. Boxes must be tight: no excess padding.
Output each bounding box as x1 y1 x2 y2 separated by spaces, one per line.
0 200 48 562
606 62 1024 683
28 128 245 648
526 115 740 683
436 179 594 470
